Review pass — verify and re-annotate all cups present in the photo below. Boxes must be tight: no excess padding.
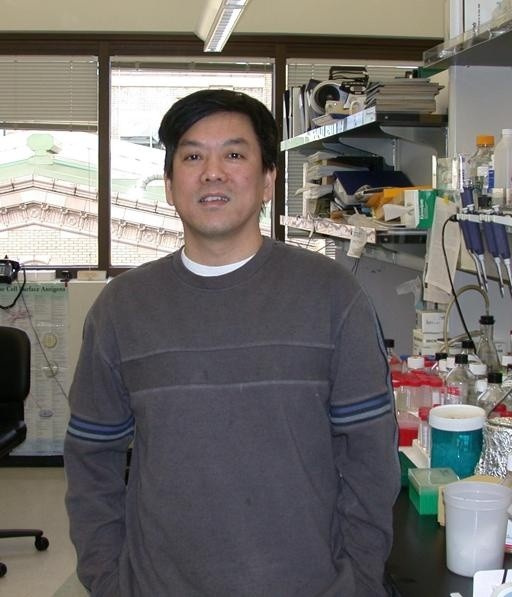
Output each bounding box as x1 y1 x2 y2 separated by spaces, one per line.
428 401 489 480
443 482 510 578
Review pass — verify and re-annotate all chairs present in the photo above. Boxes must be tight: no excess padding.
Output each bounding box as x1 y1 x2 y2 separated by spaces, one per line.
0 326 52 576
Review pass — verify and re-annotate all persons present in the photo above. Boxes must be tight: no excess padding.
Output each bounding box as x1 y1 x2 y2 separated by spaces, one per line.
61 88 401 596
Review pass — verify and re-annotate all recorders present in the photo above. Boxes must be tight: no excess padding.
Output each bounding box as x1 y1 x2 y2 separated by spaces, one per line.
308 64 369 115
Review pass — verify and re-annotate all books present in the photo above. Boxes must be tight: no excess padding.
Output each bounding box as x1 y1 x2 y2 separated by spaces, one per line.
364 80 446 115
282 87 304 140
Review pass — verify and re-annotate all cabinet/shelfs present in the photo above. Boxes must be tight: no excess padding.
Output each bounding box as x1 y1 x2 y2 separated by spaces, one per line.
279 11 512 362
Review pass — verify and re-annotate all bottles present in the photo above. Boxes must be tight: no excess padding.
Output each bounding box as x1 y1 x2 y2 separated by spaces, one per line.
384 316 512 419
464 127 512 196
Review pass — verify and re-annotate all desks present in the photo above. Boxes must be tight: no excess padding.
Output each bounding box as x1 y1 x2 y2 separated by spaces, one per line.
385 484 512 597
